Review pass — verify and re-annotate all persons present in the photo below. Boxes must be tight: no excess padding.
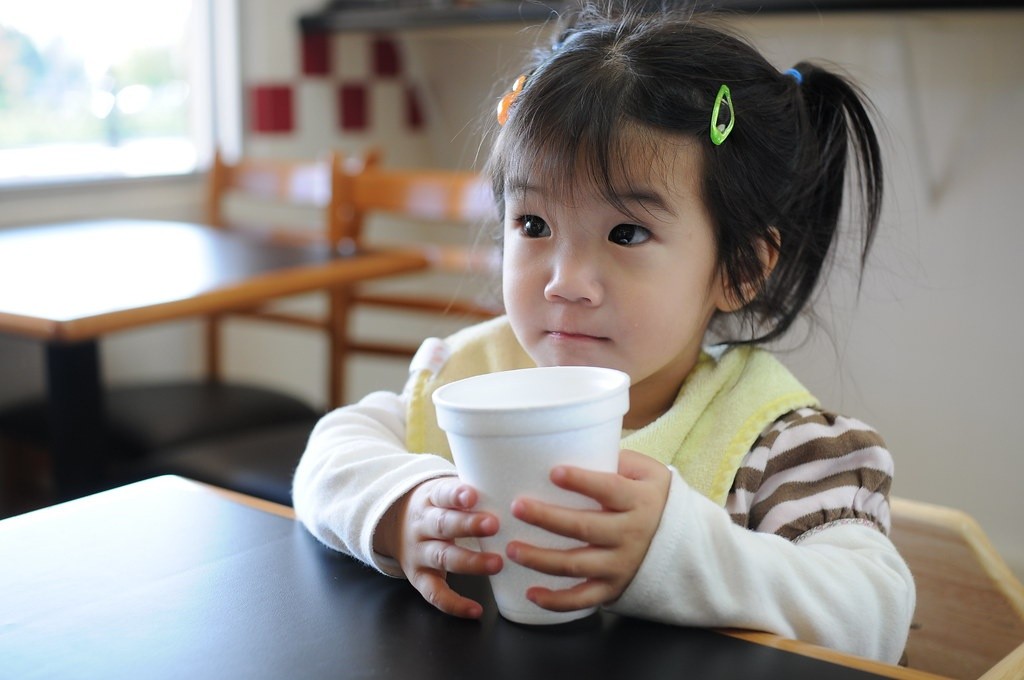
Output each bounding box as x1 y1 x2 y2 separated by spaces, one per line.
290 0 916 666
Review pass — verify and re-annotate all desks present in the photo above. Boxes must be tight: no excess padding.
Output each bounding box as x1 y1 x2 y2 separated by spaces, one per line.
0 473 948 680
0 219 424 438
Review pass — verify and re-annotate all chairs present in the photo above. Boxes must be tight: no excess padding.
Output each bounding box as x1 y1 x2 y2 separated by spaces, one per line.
196 145 505 419
878 494 1024 680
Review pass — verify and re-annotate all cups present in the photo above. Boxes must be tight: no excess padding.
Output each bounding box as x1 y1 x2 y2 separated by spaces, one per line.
431 366 631 625
